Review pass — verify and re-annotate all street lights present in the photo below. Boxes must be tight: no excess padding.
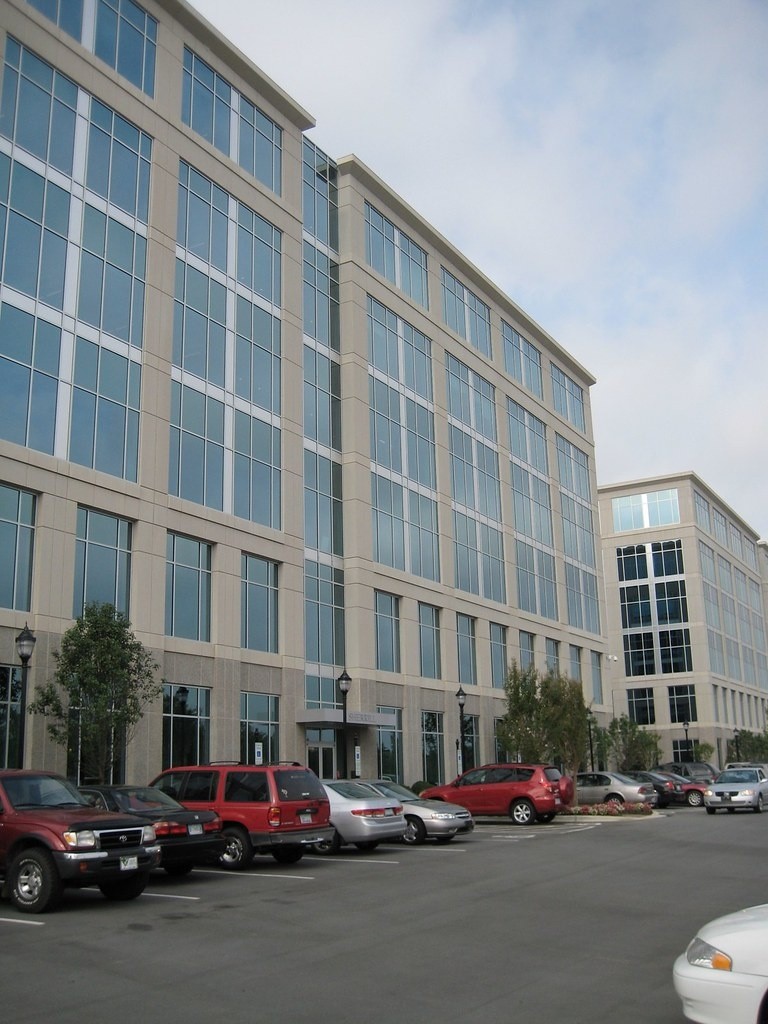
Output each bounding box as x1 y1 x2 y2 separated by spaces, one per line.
682 719 690 762
338 669 351 778
455 688 466 774
733 728 740 762
584 708 596 781
14 625 38 773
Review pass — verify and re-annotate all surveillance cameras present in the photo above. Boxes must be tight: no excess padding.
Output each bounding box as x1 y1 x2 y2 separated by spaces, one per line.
614 656 618 662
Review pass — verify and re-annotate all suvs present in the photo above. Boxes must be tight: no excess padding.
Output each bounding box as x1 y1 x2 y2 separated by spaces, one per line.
0 769 162 917
130 760 337 872
417 763 576 826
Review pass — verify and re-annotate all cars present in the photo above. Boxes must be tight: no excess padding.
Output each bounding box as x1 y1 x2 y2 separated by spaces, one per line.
358 777 477 846
651 771 708 806
702 767 768 814
568 770 660 809
39 785 223 879
672 903 768 1024
617 769 674 808
649 763 720 782
313 777 409 854
